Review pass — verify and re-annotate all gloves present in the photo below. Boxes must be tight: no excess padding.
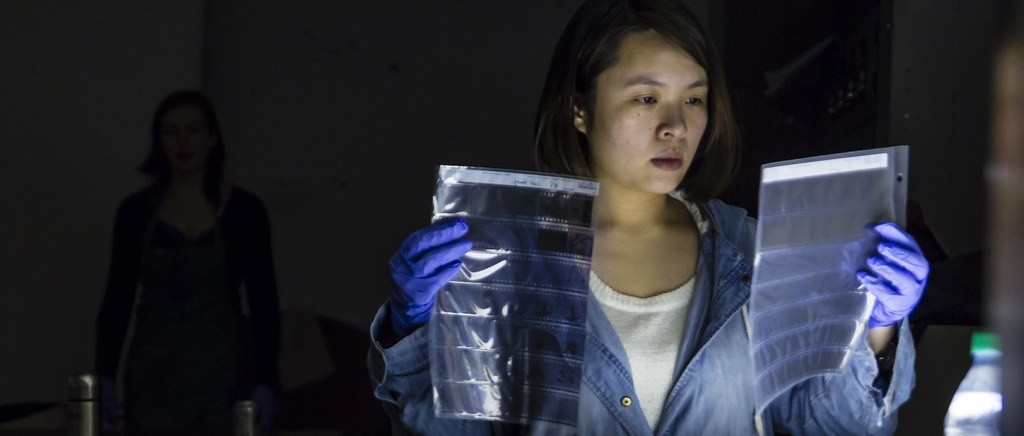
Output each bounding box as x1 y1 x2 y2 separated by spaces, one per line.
382 219 476 340
855 221 931 330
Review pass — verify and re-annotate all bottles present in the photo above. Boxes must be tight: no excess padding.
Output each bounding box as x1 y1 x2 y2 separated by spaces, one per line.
943 329 1004 436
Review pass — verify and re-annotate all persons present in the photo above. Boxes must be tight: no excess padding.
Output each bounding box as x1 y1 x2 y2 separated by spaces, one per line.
95 89 284 436
369 0 929 436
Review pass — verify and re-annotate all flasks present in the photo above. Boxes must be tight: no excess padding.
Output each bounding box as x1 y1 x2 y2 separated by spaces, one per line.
67 374 99 436
